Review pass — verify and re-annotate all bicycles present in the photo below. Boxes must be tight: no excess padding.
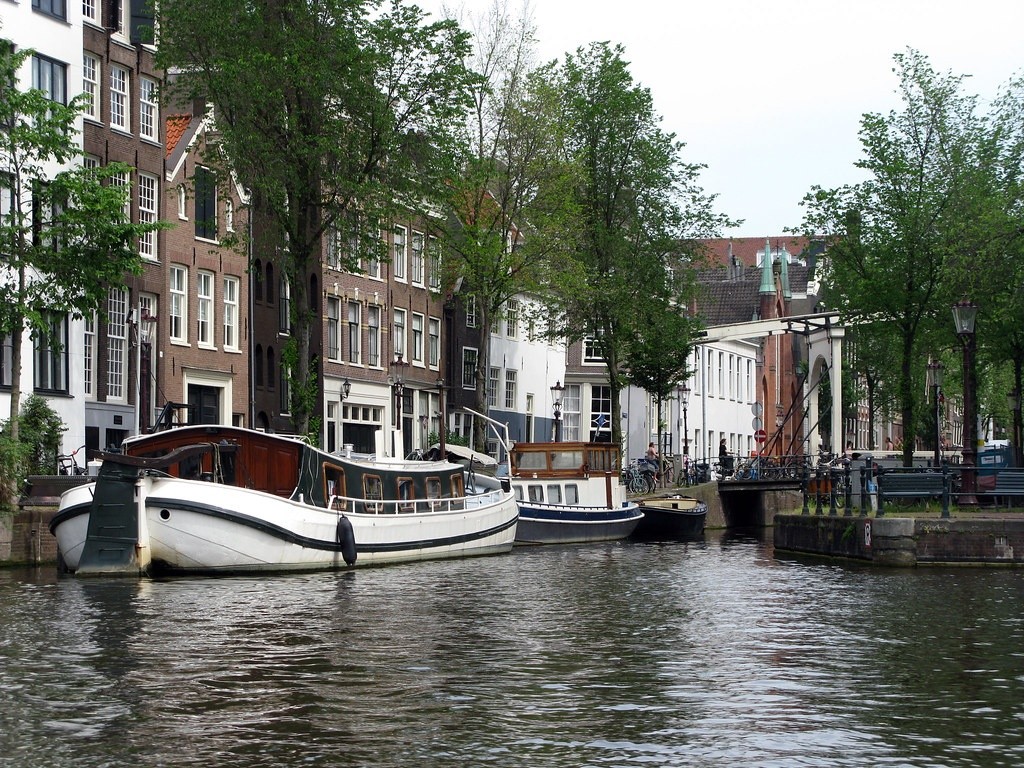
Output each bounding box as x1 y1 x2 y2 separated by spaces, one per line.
53 445 89 476
621 448 847 509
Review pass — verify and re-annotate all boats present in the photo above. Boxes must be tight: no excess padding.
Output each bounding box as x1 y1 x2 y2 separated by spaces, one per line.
45 422 520 573
628 494 709 545
390 354 408 432
496 441 644 545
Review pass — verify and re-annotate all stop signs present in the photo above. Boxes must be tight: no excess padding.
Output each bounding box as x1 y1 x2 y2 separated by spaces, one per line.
754 430 766 443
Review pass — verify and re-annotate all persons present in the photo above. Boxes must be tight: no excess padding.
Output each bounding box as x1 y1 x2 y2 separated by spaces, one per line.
718 439 735 478
886 437 894 451
648 441 660 481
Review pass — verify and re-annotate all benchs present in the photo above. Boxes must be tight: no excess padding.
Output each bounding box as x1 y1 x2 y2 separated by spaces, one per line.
881 472 953 498
984 471 1024 510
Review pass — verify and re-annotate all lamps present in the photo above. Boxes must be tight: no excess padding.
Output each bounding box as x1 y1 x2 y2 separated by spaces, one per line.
340 377 352 401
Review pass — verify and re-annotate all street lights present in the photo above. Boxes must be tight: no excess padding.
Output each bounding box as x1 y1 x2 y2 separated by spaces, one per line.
925 354 945 475
550 379 567 443
952 298 981 508
677 382 692 456
126 305 162 434
